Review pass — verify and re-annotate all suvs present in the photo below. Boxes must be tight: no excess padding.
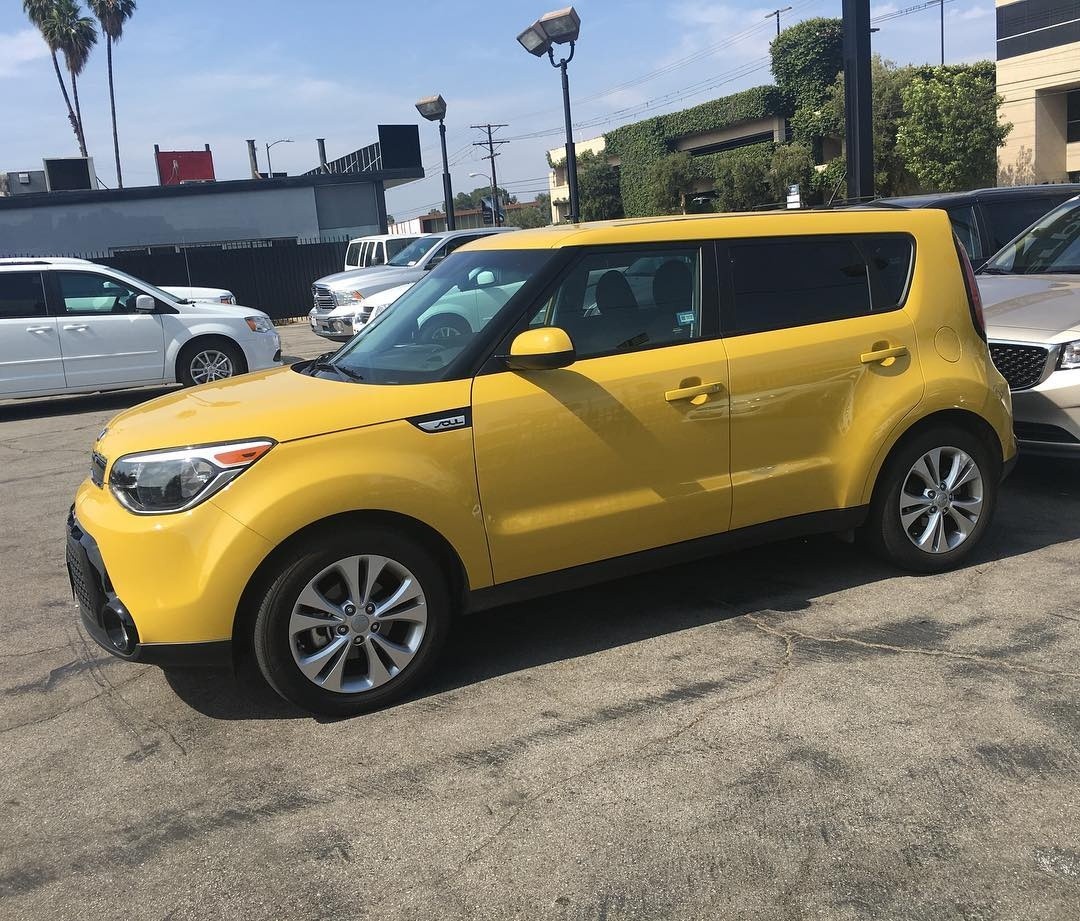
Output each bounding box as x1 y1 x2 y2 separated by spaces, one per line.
66 204 1019 716
803 183 1080 281
308 227 522 343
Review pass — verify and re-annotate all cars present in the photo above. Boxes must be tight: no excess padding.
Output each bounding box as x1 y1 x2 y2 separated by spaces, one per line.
975 195 1080 458
0 256 283 409
352 252 696 346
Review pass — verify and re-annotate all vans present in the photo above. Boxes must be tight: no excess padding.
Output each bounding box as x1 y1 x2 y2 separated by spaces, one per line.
344 234 447 273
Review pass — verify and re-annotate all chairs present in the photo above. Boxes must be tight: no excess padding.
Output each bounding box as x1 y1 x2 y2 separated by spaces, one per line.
576 260 695 360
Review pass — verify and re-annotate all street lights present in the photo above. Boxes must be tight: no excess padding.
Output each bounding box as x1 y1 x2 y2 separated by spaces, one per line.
470 173 496 226
415 94 456 231
517 6 582 223
266 138 294 178
